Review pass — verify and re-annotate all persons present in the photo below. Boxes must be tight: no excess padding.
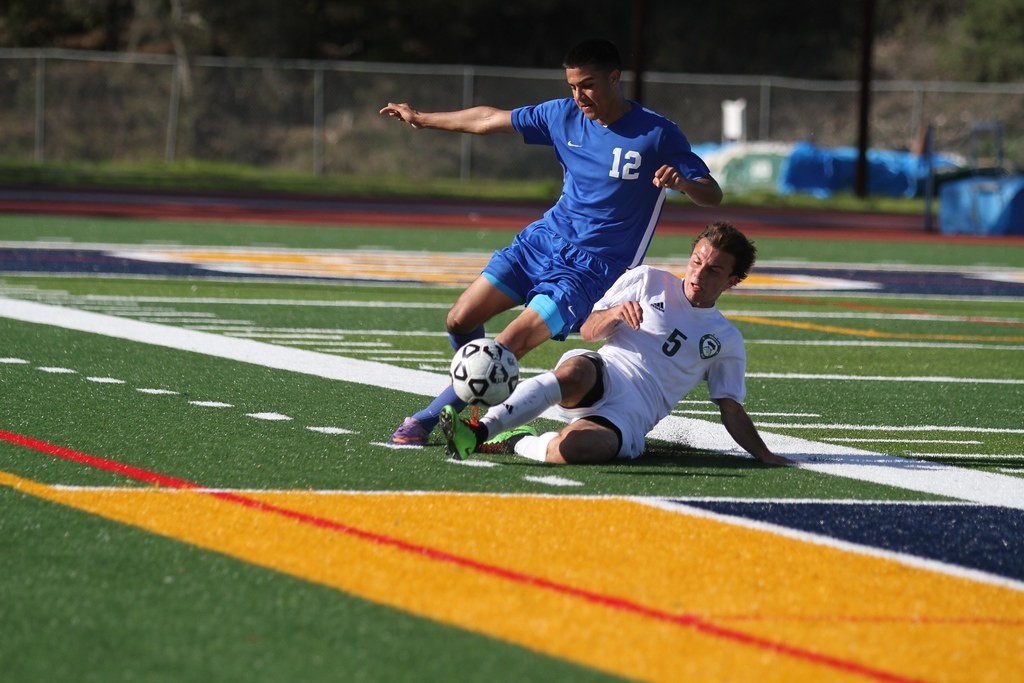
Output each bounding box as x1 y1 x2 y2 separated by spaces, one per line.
380 37 722 444
440 222 793 466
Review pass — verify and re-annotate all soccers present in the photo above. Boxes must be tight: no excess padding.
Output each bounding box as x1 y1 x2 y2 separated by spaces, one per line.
450 338 519 407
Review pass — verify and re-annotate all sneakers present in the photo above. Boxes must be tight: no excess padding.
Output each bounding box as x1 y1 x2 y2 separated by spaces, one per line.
439 404 480 460
478 425 537 455
385 416 431 448
